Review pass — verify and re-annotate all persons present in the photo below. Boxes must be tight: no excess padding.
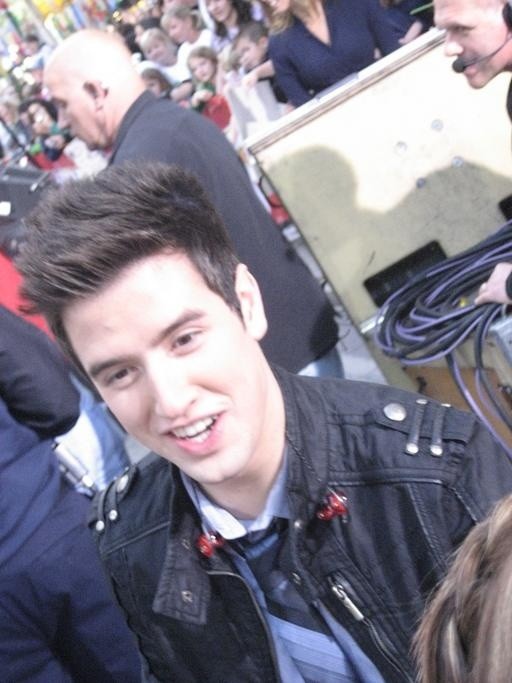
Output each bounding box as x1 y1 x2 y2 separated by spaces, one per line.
1 0 426 257
2 257 147 682
435 1 512 307
414 496 511 682
16 159 511 683
41 30 344 380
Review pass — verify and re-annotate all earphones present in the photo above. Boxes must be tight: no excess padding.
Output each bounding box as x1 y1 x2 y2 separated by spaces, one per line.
315 492 348 522
195 530 224 559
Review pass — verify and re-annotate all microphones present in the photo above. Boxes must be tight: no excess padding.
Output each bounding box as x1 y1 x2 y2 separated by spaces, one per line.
453 33 512 73
